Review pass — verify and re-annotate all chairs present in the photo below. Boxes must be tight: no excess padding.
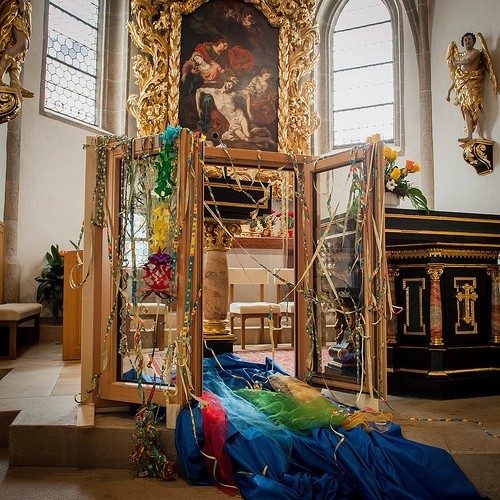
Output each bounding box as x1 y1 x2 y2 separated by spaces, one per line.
227 267 295 351
0 303 42 360
122 267 167 350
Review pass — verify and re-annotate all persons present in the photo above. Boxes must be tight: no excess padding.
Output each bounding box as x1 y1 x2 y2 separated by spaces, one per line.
0 0 35 97
446 32 485 143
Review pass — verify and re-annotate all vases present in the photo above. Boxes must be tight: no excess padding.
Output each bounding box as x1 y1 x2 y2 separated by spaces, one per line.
384 191 402 207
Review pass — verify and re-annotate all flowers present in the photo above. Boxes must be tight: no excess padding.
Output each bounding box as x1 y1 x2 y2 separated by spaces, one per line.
383 145 430 216
